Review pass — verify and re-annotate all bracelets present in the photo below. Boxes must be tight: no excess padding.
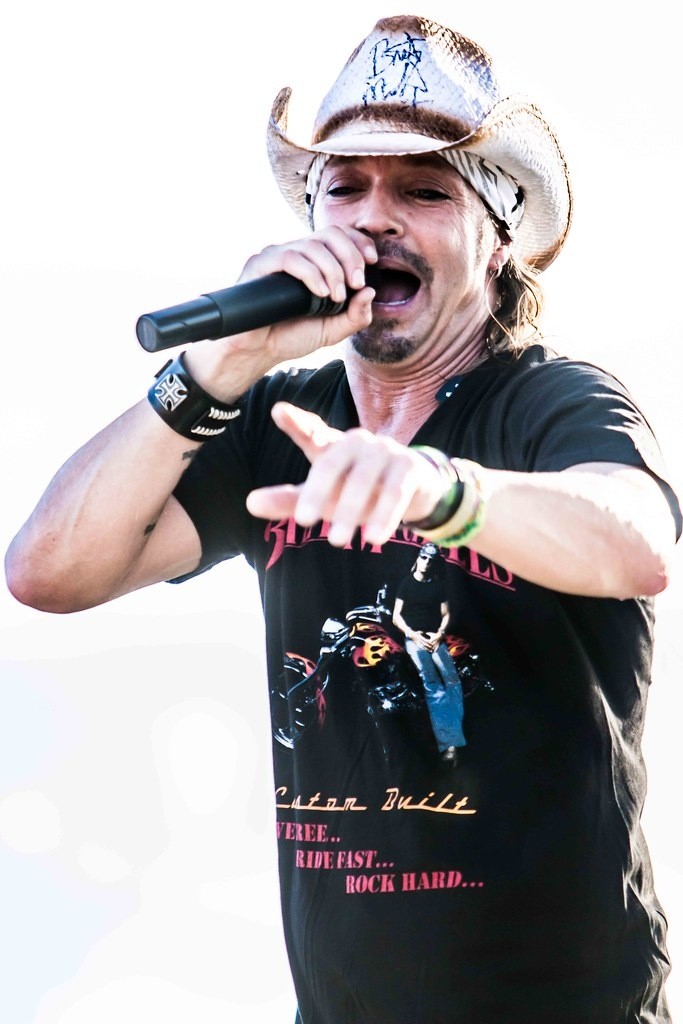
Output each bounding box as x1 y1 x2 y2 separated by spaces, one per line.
403 443 486 552
147 350 243 444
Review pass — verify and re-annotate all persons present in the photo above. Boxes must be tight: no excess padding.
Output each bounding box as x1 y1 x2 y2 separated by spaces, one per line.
6 15 683 1024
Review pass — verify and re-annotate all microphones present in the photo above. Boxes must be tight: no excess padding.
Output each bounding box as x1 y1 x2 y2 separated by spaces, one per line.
137 267 353 353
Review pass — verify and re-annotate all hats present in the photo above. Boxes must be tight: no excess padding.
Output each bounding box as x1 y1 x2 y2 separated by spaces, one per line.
266 16 571 274
